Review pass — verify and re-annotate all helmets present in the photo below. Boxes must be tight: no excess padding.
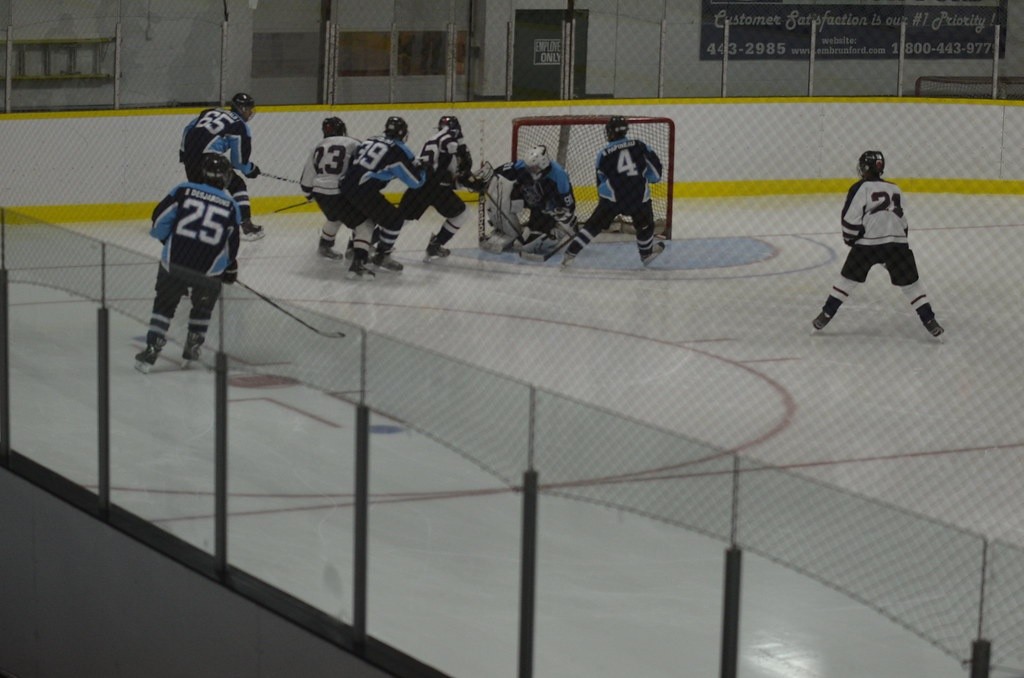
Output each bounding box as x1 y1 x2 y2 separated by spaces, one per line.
860 151 886 175
523 145 550 175
438 116 465 139
386 117 407 140
202 154 232 191
322 116 345 139
606 115 628 141
230 93 256 122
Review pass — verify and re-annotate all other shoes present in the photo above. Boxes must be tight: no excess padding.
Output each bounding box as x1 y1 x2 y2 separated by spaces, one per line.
180 332 203 369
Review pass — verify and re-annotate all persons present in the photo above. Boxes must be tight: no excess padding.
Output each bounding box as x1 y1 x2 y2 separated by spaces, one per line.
134 154 242 373
560 117 668 268
491 145 581 264
300 115 487 280
179 92 266 242
810 151 946 342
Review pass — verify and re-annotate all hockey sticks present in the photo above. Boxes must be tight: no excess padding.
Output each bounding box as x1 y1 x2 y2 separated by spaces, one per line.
274 199 313 214
518 230 578 263
235 278 345 339
484 188 522 240
259 171 300 184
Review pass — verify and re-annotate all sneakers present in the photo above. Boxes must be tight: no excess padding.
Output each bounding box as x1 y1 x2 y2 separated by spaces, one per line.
811 311 832 332
924 320 944 344
373 253 403 272
426 240 450 260
347 265 376 279
345 248 373 266
239 219 265 242
641 237 666 267
316 245 341 262
133 333 166 373
561 250 577 269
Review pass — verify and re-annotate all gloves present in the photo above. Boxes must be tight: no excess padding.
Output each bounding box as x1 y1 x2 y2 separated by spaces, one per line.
223 261 238 285
245 165 261 179
